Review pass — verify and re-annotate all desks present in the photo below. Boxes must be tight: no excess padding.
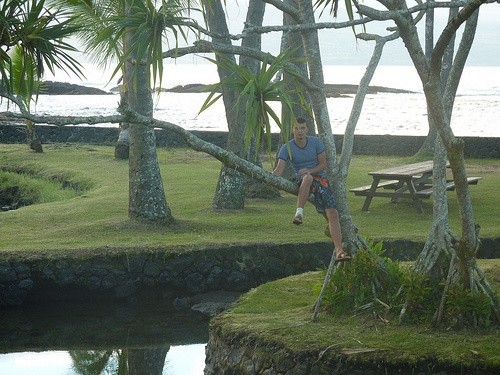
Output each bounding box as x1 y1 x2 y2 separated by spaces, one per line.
369 160 451 194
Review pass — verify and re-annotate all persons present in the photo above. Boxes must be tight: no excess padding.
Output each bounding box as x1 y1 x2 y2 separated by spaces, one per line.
271 117 352 262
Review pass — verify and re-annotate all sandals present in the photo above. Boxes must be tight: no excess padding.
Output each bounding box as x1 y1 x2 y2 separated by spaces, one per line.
335 250 352 261
292 214 303 225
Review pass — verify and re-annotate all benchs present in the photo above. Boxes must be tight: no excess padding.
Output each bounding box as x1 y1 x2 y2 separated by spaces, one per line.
348 180 400 196
413 177 481 199
